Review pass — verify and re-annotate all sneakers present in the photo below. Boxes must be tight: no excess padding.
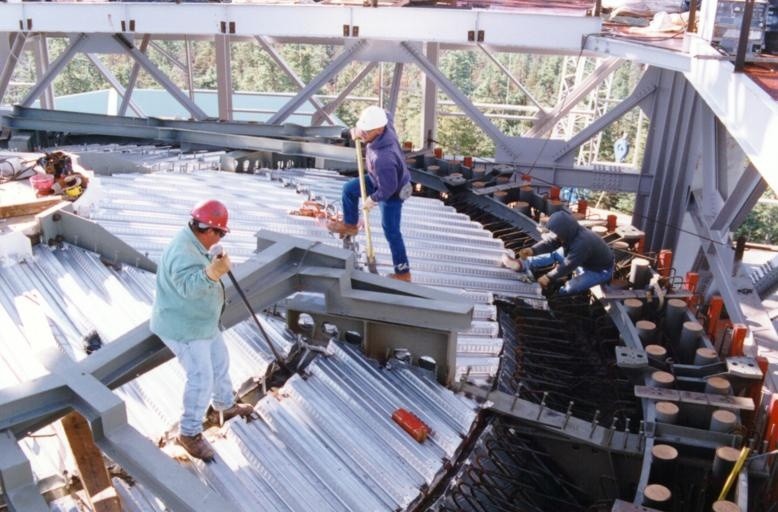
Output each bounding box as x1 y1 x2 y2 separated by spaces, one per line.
501 252 523 272
386 273 411 282
176 433 216 460
328 221 359 236
206 404 253 426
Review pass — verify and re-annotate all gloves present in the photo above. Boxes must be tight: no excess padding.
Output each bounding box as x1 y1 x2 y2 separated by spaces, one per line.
519 248 533 260
205 251 231 282
537 275 550 289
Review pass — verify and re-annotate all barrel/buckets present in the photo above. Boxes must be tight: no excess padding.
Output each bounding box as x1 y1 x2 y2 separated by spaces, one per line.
30 174 53 197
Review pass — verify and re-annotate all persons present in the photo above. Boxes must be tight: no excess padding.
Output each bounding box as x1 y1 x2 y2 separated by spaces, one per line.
503 211 617 298
329 104 412 282
149 199 253 460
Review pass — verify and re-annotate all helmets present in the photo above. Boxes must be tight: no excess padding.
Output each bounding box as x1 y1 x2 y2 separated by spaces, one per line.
31 174 61 198
190 200 228 232
356 106 388 131
